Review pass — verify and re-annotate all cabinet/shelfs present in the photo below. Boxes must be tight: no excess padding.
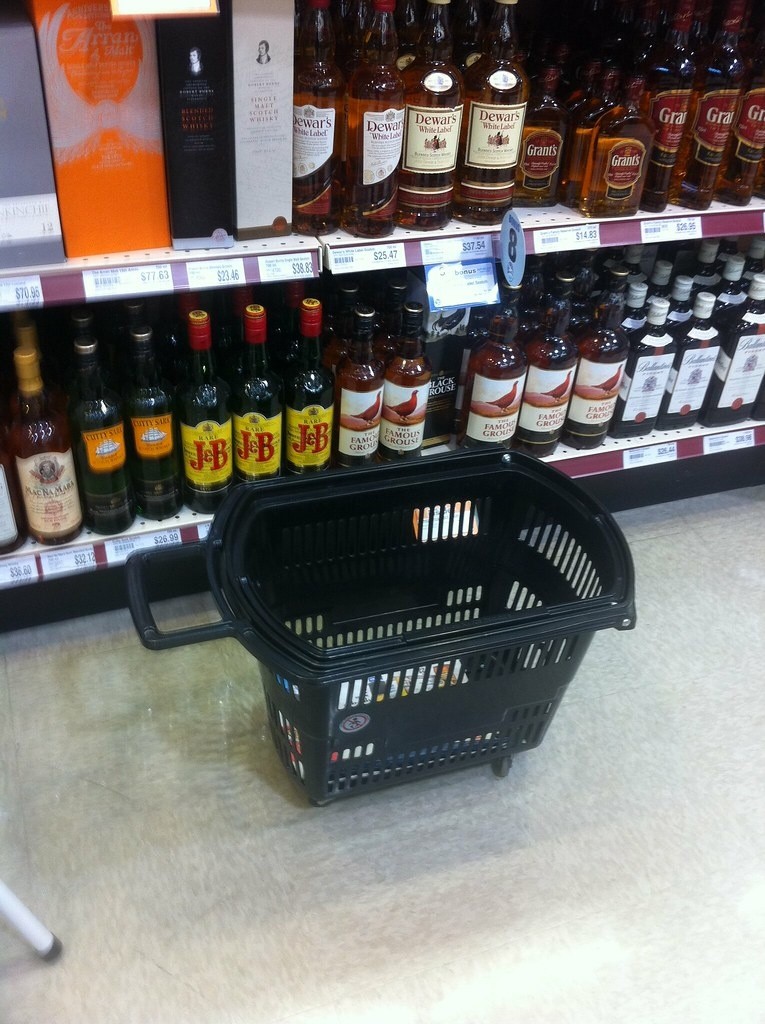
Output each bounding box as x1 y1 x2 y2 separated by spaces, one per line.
0 194 765 637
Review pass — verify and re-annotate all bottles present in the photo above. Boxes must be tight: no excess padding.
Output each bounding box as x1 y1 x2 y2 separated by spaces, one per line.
293 0 765 237
0 240 765 553
